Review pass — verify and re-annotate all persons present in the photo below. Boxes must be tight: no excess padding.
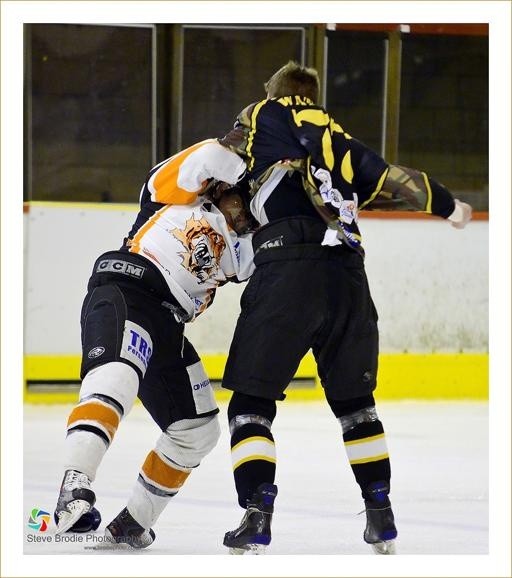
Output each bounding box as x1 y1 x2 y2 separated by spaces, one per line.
54 126 257 548
218 63 472 547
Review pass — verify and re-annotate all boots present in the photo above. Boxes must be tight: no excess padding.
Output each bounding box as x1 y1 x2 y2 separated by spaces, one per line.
105 506 156 549
223 484 278 547
362 485 397 544
54 469 97 525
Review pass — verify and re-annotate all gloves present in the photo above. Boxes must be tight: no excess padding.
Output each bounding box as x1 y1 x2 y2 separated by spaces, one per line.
448 199 472 229
